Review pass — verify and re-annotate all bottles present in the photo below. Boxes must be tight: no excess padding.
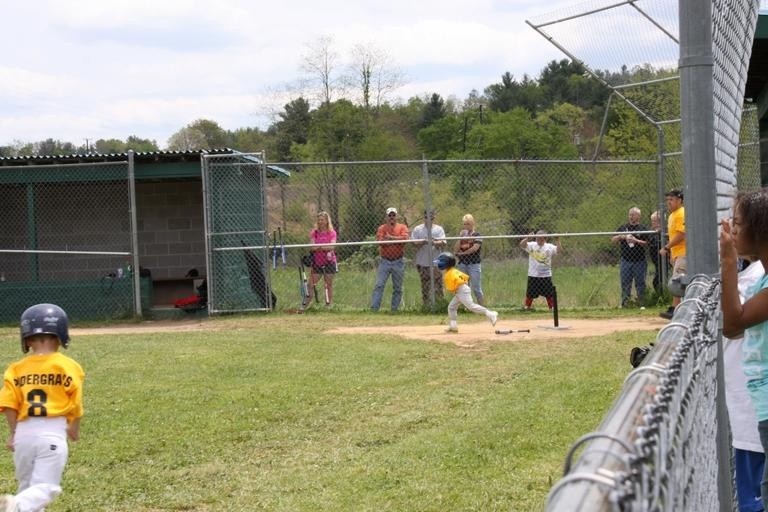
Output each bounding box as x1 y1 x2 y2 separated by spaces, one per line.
625 230 634 248
327 252 332 261
117 262 132 280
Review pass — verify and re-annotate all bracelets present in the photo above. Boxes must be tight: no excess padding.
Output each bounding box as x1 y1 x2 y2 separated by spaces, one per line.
664 245 669 251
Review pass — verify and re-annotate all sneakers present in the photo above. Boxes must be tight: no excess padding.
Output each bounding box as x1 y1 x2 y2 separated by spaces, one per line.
443 327 459 334
0 492 19 512
659 306 676 319
490 311 499 327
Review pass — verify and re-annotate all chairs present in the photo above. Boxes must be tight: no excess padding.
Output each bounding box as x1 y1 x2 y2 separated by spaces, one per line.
171 277 207 326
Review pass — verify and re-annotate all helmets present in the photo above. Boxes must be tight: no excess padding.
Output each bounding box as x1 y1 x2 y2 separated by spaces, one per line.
20 302 70 353
432 252 456 271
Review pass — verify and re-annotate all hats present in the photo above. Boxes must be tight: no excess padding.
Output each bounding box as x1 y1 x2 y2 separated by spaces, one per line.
664 188 683 200
385 207 398 216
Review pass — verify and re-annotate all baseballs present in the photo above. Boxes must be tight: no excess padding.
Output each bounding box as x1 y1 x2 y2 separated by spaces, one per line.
640 306 645 311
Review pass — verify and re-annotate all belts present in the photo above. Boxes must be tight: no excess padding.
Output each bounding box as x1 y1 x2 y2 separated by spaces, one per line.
381 256 403 262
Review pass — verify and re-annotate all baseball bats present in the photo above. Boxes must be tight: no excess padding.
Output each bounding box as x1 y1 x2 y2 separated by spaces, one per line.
278 226 286 267
272 230 277 271
298 256 330 306
494 330 530 335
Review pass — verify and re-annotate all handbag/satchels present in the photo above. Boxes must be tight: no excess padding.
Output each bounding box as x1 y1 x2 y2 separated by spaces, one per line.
302 252 315 267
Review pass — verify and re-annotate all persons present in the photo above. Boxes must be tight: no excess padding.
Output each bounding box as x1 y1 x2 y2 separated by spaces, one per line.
518 229 563 310
453 214 484 306
303 211 339 309
370 207 409 311
410 209 447 304
0 302 85 512
657 187 687 318
718 189 768 512
610 206 649 308
436 251 499 333
645 210 670 296
